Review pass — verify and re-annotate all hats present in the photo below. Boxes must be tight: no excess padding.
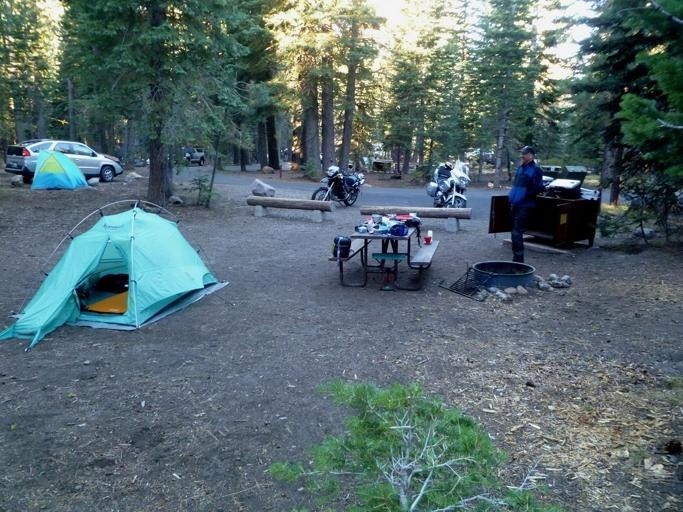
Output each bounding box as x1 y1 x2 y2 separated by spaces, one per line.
518 145 536 156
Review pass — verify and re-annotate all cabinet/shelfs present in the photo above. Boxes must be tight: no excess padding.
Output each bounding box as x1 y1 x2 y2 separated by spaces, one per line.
488 187 602 248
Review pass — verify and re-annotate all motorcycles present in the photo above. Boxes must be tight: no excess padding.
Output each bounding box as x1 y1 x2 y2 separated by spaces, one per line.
310 163 364 208
423 154 470 210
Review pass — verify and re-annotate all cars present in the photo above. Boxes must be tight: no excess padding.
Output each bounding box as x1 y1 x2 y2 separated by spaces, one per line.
461 146 496 166
132 147 206 166
98 152 119 165
5 138 123 181
319 153 422 177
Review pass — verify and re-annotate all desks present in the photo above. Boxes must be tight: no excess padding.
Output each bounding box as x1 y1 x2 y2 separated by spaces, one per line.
348 217 423 292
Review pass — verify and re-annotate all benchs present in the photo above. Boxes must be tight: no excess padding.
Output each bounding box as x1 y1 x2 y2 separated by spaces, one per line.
358 204 472 234
326 237 373 290
408 238 441 290
246 196 334 223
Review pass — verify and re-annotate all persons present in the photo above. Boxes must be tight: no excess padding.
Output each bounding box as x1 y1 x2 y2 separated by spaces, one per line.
508 145 543 262
434 162 453 183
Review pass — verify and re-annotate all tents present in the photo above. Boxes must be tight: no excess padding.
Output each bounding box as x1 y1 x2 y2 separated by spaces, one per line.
0 200 218 349
31 149 88 191
